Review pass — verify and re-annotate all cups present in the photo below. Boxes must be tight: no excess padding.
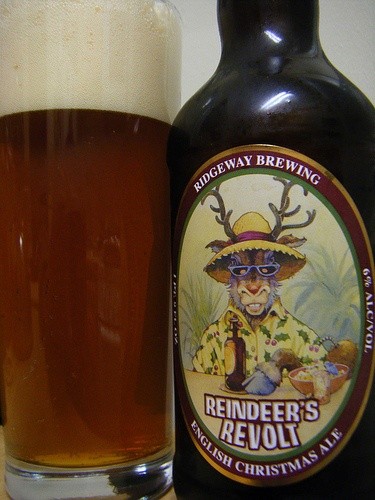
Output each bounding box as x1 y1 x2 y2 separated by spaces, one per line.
0 0 183 500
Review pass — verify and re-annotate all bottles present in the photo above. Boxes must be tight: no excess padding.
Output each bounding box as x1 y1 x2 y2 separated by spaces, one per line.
174 0 375 499
